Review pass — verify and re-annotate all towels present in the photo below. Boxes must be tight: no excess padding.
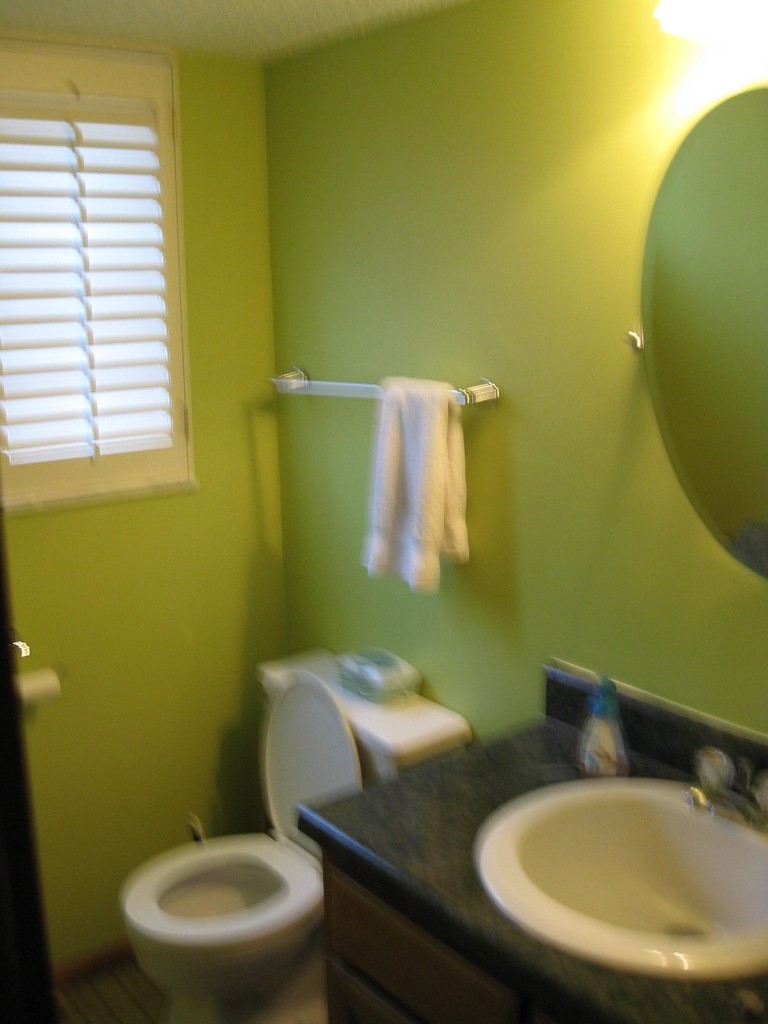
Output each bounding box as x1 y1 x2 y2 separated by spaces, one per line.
359 377 470 597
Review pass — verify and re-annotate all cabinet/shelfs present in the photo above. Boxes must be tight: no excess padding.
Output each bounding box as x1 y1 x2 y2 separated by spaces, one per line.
315 840 587 1023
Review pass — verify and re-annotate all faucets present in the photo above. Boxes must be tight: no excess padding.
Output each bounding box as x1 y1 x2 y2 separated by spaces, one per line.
683 786 768 831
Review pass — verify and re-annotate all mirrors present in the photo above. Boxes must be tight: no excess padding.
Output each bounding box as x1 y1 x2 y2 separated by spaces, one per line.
629 84 768 578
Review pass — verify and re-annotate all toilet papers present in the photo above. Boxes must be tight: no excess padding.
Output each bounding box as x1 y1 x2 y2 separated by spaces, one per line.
13 667 61 706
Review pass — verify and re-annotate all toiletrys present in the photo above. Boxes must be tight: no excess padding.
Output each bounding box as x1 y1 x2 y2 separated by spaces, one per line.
577 675 628 777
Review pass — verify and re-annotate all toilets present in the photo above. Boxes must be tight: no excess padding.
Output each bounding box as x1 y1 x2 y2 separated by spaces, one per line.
119 647 473 1024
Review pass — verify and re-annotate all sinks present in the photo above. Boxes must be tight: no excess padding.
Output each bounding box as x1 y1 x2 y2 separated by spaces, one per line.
471 777 768 981
